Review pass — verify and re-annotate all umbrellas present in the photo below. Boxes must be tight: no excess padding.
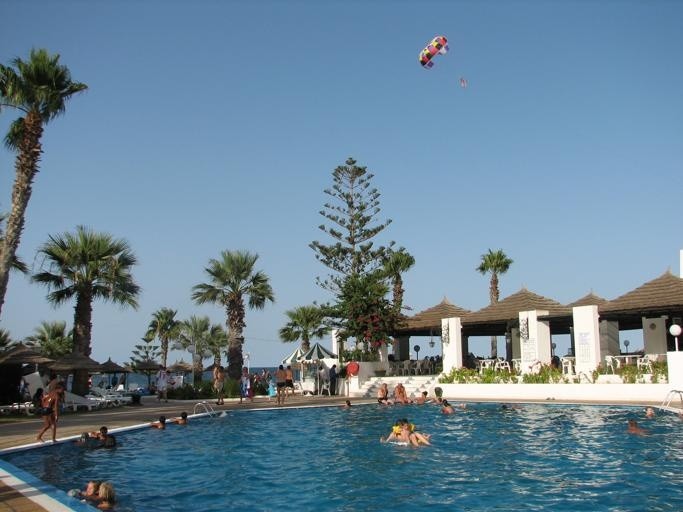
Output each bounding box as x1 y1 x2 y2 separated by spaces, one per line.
281 349 305 381
296 342 338 396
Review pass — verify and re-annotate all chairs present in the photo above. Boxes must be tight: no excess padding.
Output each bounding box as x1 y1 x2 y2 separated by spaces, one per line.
292 378 332 396
388 353 661 376
1 372 133 416
